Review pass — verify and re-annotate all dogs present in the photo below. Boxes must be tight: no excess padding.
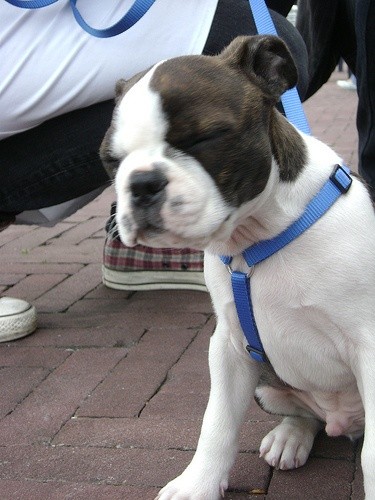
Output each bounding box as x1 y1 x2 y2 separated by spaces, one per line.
100 36 373 500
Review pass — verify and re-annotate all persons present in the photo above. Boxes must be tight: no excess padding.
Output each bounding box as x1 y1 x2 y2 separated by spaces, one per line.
2 0 308 350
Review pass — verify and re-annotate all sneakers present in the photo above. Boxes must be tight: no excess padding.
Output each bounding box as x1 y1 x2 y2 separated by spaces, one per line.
100 206 208 293
0 295 40 344
